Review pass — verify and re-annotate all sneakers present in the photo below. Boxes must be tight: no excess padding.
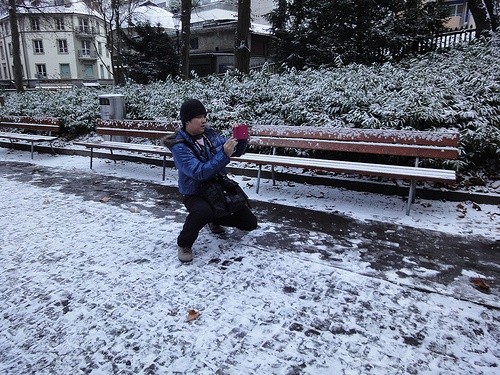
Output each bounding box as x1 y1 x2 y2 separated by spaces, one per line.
208 222 225 233
178 245 193 263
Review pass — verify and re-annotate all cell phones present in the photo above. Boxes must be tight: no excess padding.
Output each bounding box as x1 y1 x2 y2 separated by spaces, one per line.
233 125 248 139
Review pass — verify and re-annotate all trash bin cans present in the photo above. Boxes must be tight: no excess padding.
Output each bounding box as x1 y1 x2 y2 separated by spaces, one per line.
97 94 129 143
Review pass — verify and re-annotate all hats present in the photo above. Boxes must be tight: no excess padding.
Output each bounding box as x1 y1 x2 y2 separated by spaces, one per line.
180 99 206 124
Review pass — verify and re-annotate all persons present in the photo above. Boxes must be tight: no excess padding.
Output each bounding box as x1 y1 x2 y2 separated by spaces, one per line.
163 97 257 262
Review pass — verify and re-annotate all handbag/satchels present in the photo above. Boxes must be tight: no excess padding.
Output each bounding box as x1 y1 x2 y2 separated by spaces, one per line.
200 174 251 219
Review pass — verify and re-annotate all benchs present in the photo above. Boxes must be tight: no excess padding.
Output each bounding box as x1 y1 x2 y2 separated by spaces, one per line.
72 119 183 182
0 115 61 159
229 125 459 216
